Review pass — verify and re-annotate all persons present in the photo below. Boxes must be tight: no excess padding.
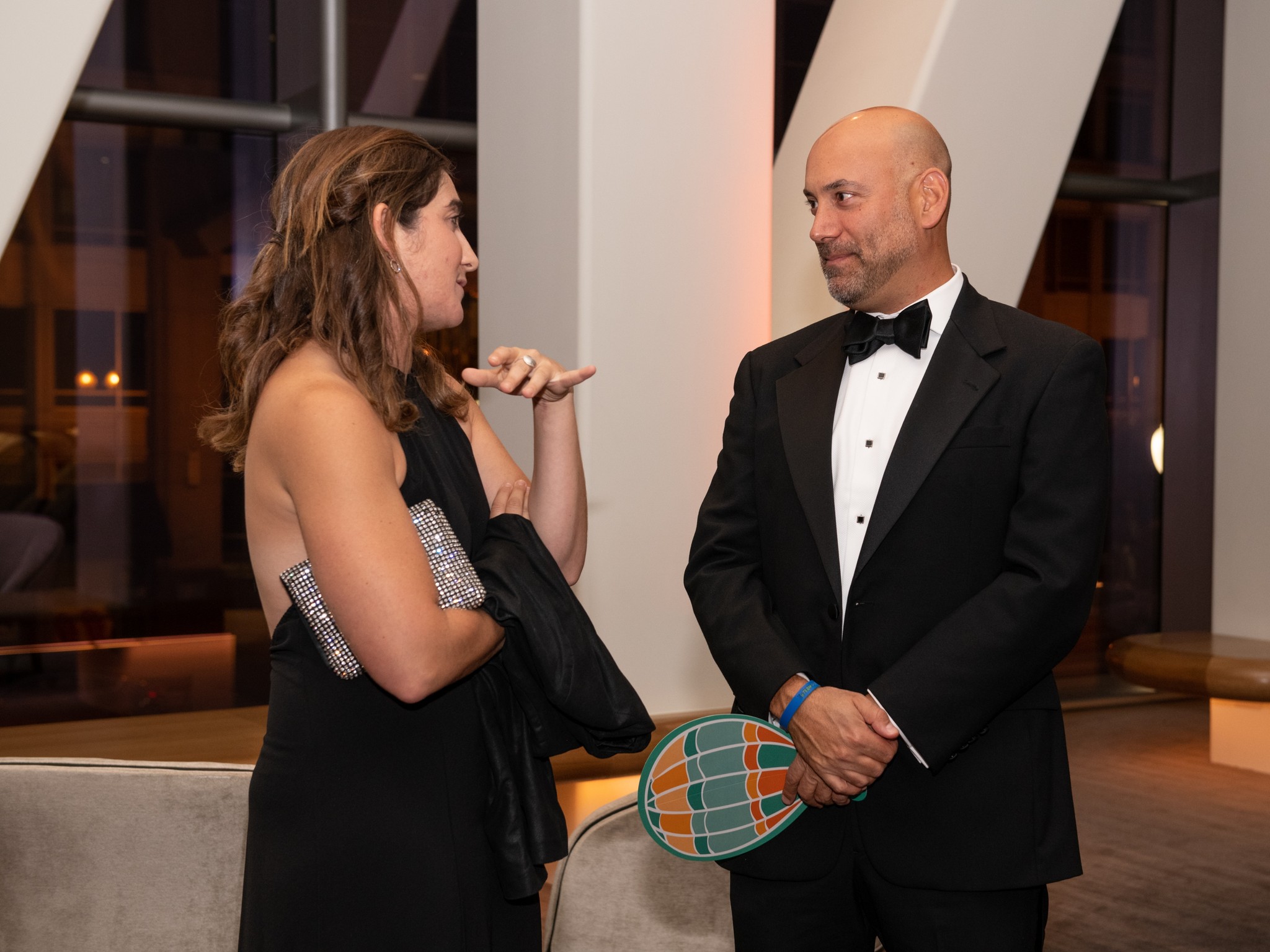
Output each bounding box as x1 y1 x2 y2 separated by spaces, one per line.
199 122 599 952
681 108 1100 952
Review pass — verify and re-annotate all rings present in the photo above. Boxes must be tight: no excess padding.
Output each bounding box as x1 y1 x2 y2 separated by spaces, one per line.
515 355 537 368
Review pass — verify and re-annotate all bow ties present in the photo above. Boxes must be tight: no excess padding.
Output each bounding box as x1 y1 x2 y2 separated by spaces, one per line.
841 298 933 365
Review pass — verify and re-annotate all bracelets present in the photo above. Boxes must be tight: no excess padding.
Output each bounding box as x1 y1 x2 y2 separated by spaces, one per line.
780 680 819 732
